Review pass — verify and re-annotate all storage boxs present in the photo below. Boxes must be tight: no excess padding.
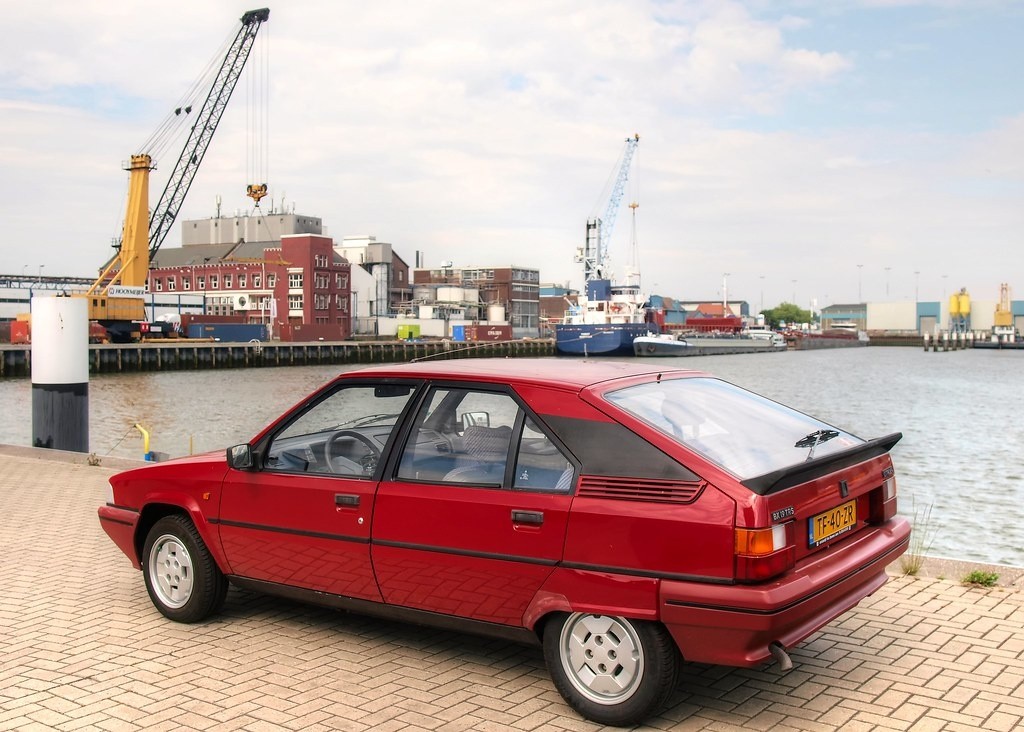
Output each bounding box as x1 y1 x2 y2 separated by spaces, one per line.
182 312 268 342
453 325 515 344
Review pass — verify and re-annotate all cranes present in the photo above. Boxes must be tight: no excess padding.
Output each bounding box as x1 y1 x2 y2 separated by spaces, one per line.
574 133 642 279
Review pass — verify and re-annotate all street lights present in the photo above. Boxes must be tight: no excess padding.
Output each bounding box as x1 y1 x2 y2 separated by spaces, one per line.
39 264 45 289
857 264 864 303
351 290 359 335
914 271 919 302
723 272 731 319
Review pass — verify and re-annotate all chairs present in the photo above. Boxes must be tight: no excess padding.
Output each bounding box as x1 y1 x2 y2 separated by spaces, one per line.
441 422 514 484
526 400 730 491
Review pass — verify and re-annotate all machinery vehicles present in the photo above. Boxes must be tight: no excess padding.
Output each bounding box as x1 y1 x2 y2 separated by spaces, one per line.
53 4 293 346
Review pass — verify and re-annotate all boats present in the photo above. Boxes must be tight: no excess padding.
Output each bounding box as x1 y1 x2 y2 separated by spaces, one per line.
786 329 861 350
548 274 744 355
633 328 788 357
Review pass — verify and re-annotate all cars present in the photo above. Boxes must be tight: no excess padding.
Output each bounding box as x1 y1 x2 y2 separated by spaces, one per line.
99 359 913 727
